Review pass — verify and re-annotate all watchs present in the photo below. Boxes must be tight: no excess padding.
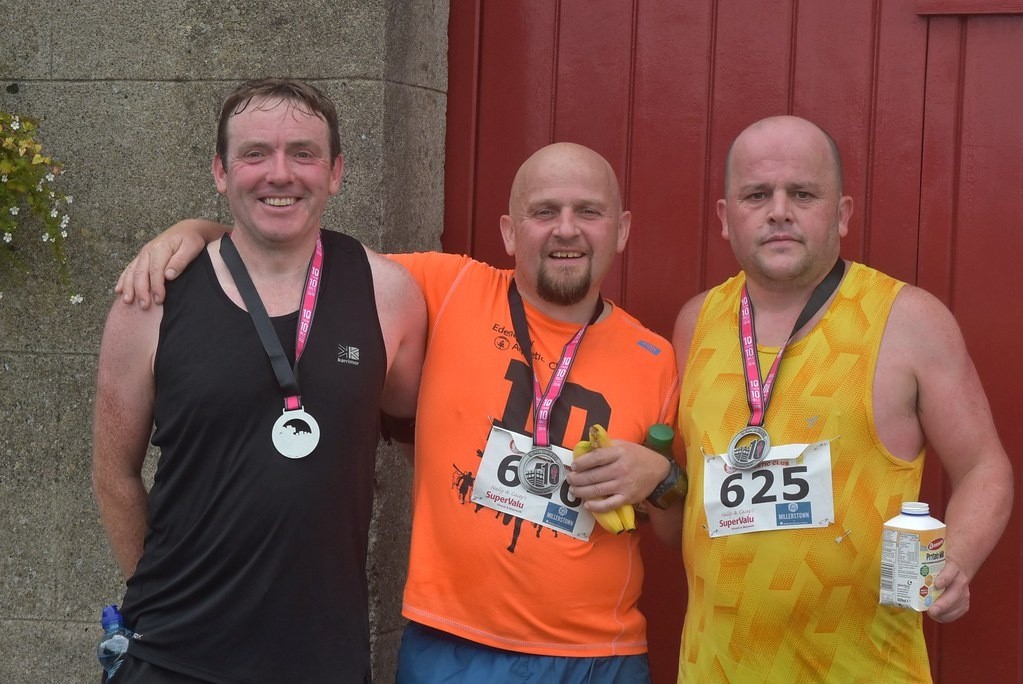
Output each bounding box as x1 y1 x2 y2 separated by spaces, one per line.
646 462 688 511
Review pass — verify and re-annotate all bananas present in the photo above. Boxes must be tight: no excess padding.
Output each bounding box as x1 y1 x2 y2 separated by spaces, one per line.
572 425 636 535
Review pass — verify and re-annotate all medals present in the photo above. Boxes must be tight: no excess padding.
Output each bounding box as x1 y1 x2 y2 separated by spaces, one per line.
272 406 320 459
517 449 564 496
728 426 770 471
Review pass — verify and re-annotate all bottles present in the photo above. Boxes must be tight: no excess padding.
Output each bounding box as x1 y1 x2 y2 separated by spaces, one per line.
97 605 134 679
878 501 946 611
641 424 674 462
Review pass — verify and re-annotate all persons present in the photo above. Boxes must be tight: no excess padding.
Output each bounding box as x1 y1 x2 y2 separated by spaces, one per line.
671 116 1014 684
114 141 689 684
93 77 428 684
